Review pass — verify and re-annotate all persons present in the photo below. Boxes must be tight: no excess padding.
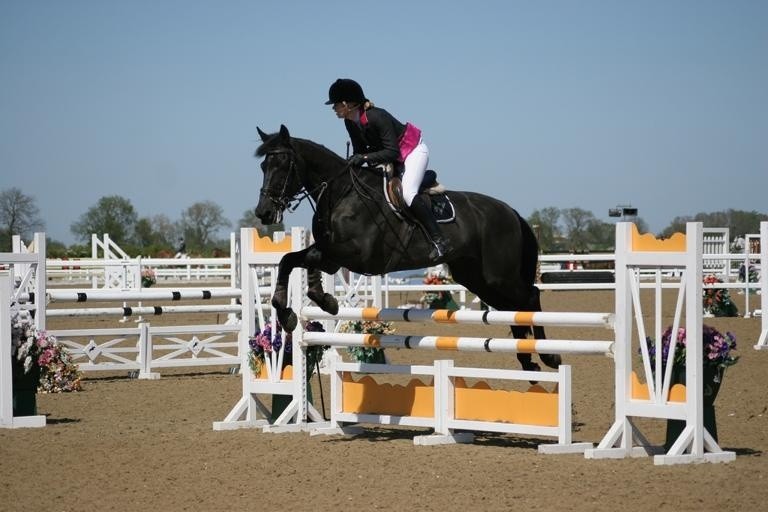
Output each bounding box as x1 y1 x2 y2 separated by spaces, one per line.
325 79 454 260
172 237 186 259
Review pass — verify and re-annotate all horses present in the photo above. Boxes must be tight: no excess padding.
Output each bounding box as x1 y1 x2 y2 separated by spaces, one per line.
253 123 562 386
157 248 231 279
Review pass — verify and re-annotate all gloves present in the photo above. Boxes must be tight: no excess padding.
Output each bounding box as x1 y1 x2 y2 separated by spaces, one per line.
351 153 365 168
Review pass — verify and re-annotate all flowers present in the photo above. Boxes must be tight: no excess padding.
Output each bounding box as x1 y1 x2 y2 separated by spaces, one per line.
738 261 761 294
338 319 396 365
141 268 157 287
246 317 331 375
11 313 86 392
419 270 459 310
637 322 741 403
702 273 738 317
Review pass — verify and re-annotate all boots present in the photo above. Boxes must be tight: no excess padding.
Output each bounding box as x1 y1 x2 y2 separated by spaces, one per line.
407 194 454 260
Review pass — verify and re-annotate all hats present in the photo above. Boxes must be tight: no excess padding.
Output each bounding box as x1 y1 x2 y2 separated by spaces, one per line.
324 78 364 105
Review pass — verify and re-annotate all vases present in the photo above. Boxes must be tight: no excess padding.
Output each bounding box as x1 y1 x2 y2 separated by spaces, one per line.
271 382 313 423
12 355 38 416
663 404 719 453
